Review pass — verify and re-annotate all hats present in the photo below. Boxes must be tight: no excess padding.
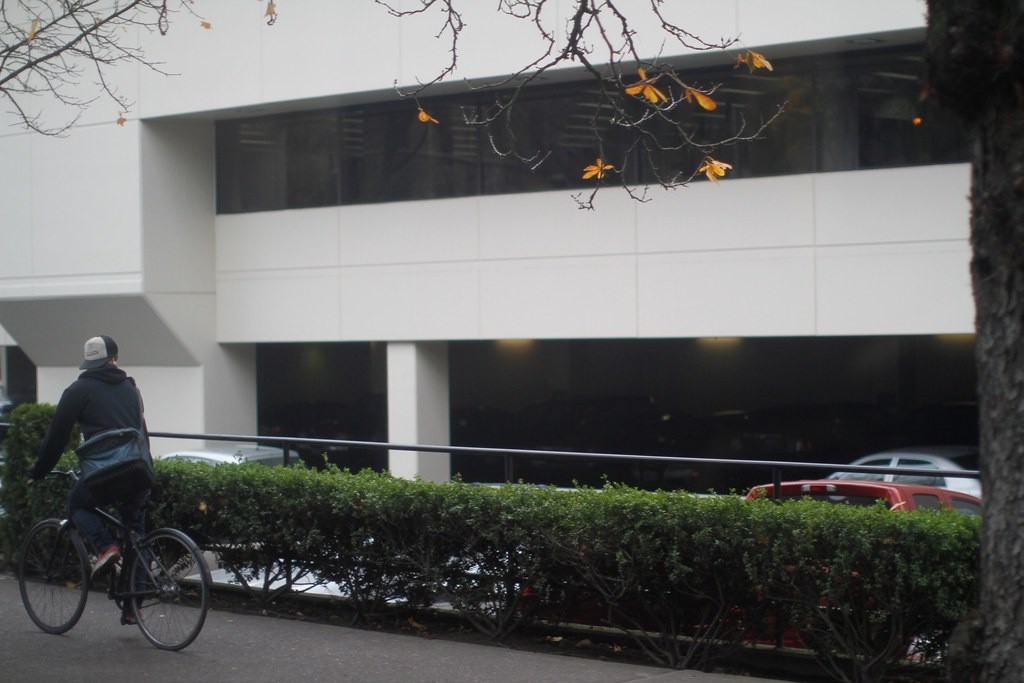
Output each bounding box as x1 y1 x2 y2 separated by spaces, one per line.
79 335 118 370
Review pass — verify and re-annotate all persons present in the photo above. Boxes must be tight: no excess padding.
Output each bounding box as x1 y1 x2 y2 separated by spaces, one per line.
29 335 153 624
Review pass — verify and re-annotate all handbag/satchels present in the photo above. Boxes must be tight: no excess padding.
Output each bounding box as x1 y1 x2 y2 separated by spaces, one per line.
76 427 154 503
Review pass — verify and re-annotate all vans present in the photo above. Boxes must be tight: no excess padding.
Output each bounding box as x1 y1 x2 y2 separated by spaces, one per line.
743 478 984 659
156 442 301 468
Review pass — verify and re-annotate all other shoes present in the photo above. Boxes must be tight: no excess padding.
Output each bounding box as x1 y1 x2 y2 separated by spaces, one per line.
121 605 145 624
91 546 121 581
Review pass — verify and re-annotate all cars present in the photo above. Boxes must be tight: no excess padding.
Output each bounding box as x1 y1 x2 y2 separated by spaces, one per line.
820 451 983 497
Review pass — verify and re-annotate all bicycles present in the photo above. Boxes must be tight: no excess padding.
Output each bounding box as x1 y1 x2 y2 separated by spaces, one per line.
17 467 213 651
